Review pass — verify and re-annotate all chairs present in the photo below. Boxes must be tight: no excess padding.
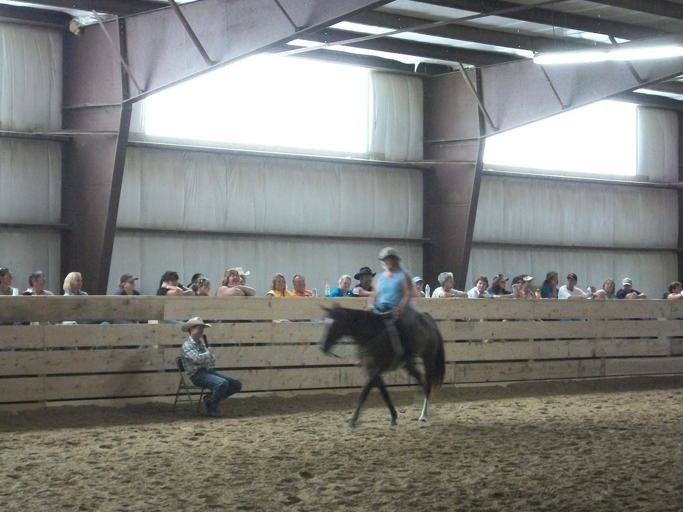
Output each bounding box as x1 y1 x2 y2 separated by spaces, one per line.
170 353 212 418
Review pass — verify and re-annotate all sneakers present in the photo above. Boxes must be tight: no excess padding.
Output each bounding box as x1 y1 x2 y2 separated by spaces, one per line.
202 396 223 417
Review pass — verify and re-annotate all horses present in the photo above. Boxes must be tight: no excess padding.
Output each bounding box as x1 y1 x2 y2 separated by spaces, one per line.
314 300 446 431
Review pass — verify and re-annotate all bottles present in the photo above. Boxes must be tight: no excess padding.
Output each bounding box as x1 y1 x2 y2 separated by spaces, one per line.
585 284 592 300
424 284 431 299
534 289 542 299
312 288 318 296
322 283 330 296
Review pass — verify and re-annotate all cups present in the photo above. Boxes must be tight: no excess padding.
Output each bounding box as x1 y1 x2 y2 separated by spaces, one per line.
512 287 519 297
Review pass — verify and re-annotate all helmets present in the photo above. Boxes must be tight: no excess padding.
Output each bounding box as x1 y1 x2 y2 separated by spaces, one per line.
377 246 404 262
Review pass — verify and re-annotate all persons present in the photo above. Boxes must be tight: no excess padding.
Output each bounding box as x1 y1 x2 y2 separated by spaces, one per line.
179 316 242 416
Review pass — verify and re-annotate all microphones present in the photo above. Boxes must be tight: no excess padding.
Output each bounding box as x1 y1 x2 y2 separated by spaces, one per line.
203 334 208 348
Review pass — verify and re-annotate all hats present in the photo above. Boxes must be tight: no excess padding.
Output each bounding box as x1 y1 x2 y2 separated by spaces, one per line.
523 276 533 281
493 273 509 283
354 266 377 281
411 276 424 284
120 273 139 283
622 278 634 285
567 273 577 280
181 316 211 332
227 267 250 276
